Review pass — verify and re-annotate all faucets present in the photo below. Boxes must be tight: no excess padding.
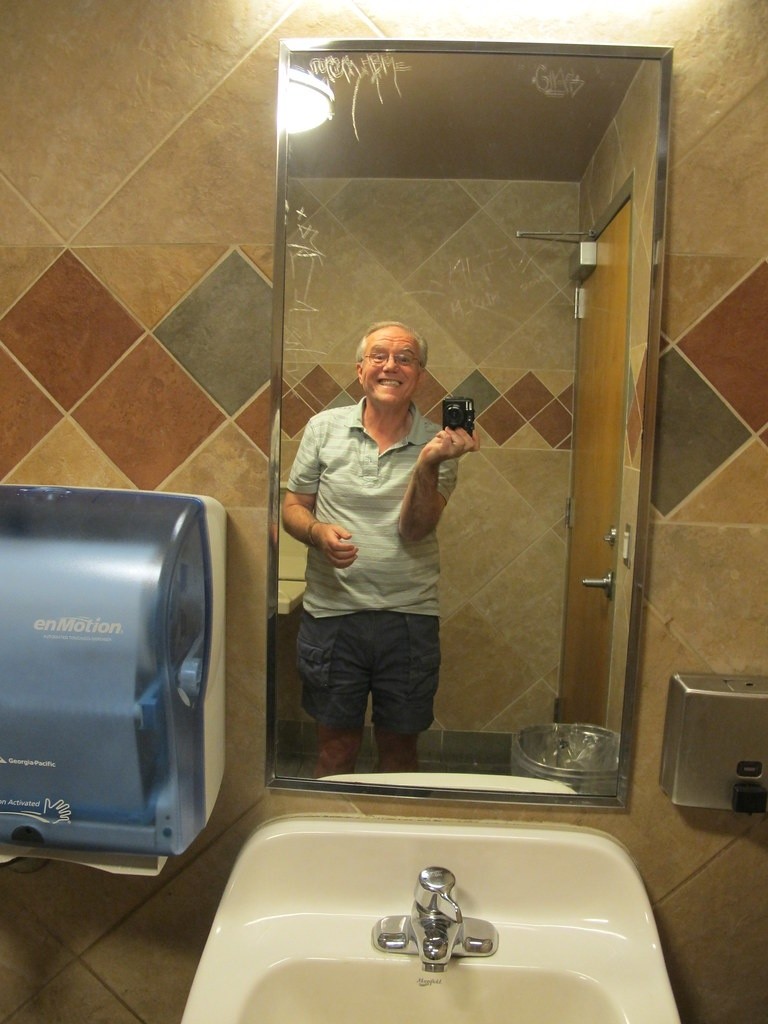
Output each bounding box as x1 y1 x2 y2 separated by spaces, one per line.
409 866 468 971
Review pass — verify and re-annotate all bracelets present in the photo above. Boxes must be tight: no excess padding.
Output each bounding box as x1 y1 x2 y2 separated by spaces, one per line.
308 521 321 545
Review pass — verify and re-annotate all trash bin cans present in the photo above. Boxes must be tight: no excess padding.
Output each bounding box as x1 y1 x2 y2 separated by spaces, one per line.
511 719 620 794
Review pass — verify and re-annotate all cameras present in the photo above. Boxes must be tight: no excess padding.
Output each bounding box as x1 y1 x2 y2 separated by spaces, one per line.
442 397 476 437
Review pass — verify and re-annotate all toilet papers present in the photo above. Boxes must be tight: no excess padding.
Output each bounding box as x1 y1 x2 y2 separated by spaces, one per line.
0 536 191 880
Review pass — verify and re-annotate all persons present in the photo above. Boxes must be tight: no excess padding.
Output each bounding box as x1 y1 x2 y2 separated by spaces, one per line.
283 322 481 779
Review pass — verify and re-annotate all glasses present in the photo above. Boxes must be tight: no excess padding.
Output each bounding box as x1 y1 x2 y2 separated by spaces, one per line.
361 351 422 366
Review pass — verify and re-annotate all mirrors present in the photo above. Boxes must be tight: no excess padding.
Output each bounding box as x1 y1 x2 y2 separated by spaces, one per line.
263 36 672 810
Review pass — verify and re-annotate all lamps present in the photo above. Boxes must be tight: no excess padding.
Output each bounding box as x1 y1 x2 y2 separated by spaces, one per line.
286 64 336 135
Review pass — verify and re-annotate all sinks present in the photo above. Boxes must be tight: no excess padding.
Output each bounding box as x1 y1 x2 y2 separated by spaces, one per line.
180 815 680 1023
315 771 578 798
